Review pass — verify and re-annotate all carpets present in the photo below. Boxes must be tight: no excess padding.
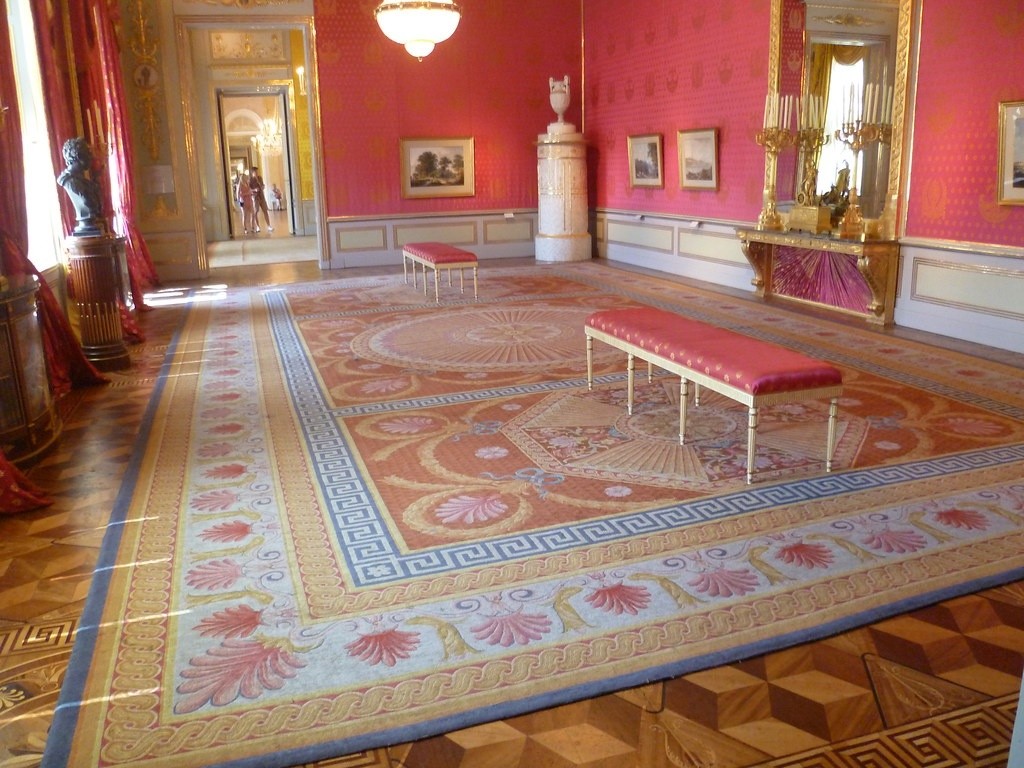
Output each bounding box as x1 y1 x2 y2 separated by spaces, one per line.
38 262 1024 767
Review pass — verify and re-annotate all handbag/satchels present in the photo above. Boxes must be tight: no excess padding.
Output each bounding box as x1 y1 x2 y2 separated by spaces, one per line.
240 202 244 206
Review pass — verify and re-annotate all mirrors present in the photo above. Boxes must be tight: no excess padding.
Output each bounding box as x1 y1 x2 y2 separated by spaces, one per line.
746 0 924 242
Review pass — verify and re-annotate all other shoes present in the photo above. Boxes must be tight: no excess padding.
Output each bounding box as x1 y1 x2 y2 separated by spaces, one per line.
268 225 274 231
251 229 256 233
257 225 261 232
245 229 248 234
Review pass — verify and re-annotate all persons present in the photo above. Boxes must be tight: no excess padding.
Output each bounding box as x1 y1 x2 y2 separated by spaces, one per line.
237 167 283 234
56 138 102 237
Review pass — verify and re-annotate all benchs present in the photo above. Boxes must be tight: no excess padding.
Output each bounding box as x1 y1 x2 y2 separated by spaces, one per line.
583 305 843 486
401 241 479 303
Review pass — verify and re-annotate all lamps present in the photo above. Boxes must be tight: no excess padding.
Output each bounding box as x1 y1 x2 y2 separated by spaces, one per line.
251 96 283 157
372 0 462 65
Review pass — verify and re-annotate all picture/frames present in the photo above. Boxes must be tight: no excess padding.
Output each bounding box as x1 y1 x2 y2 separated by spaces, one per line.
626 133 664 190
398 135 475 197
676 127 719 192
996 99 1024 206
